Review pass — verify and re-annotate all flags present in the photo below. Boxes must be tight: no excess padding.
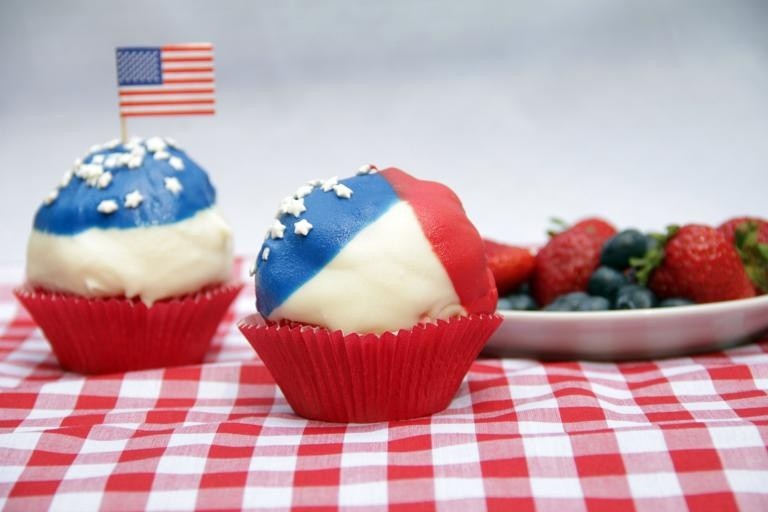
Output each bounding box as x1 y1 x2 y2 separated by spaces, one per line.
116 44 215 116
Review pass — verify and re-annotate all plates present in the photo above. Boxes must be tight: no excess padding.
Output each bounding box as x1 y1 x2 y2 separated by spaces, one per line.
484 293 767 358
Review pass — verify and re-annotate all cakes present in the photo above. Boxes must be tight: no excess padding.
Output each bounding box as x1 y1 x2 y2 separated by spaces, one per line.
12 135 246 374
237 165 505 423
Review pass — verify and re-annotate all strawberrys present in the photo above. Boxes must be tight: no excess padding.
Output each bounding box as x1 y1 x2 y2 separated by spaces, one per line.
485 217 619 298
629 223 756 305
716 216 768 286
